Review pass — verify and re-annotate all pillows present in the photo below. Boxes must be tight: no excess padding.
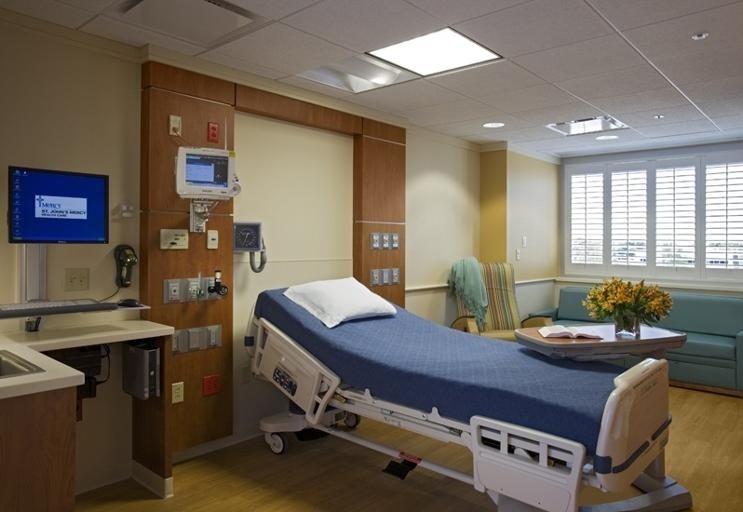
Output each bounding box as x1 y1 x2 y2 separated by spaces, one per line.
283 276 397 329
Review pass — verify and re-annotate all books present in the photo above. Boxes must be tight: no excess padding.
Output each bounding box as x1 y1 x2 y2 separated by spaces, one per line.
537 325 602 340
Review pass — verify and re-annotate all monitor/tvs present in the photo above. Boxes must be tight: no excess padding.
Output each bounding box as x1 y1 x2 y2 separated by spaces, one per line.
176 146 236 200
7 163 109 245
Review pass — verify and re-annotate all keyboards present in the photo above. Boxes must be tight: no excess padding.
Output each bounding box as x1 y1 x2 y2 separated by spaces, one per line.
0 298 97 311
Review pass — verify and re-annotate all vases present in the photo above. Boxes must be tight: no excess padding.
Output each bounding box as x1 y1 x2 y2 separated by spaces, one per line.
614 310 641 337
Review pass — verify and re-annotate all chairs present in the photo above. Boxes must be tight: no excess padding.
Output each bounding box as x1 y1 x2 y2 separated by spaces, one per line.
450 262 554 341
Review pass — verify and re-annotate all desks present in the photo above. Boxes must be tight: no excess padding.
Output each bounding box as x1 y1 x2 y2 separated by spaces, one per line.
0 318 175 512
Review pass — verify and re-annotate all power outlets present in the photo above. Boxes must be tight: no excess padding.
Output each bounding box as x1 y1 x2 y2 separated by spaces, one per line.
170 115 182 136
64 268 90 290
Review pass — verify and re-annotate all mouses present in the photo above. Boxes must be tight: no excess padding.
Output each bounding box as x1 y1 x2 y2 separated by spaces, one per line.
117 298 142 307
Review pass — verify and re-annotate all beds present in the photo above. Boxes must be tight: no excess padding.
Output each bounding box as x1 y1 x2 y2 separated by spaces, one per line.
246 276 691 512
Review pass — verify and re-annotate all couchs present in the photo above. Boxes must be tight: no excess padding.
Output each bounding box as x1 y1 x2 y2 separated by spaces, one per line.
529 284 743 397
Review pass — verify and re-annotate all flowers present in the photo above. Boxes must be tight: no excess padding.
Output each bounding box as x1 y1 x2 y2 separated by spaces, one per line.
584 278 671 322
